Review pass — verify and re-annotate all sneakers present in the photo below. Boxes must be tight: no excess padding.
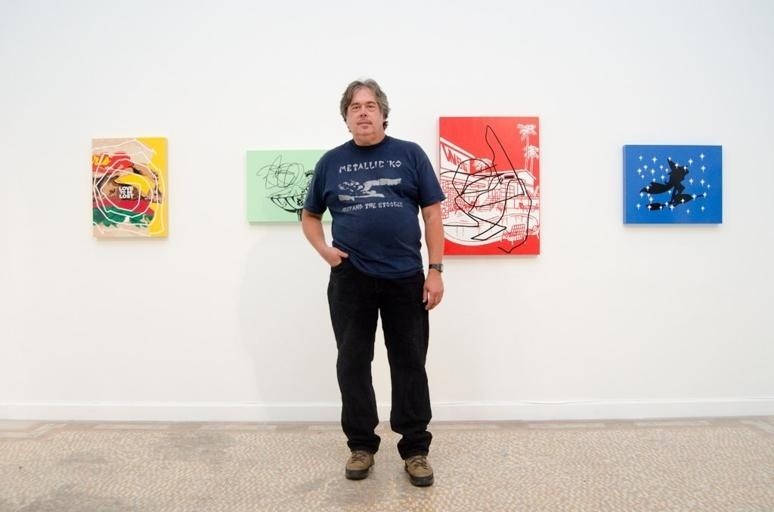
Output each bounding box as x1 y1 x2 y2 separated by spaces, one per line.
405 455 433 485
346 450 374 479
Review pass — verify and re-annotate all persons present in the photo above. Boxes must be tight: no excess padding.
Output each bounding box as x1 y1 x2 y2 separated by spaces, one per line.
301 77 445 487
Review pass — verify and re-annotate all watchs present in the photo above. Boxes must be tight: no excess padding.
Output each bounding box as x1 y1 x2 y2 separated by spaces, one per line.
428 263 444 272
